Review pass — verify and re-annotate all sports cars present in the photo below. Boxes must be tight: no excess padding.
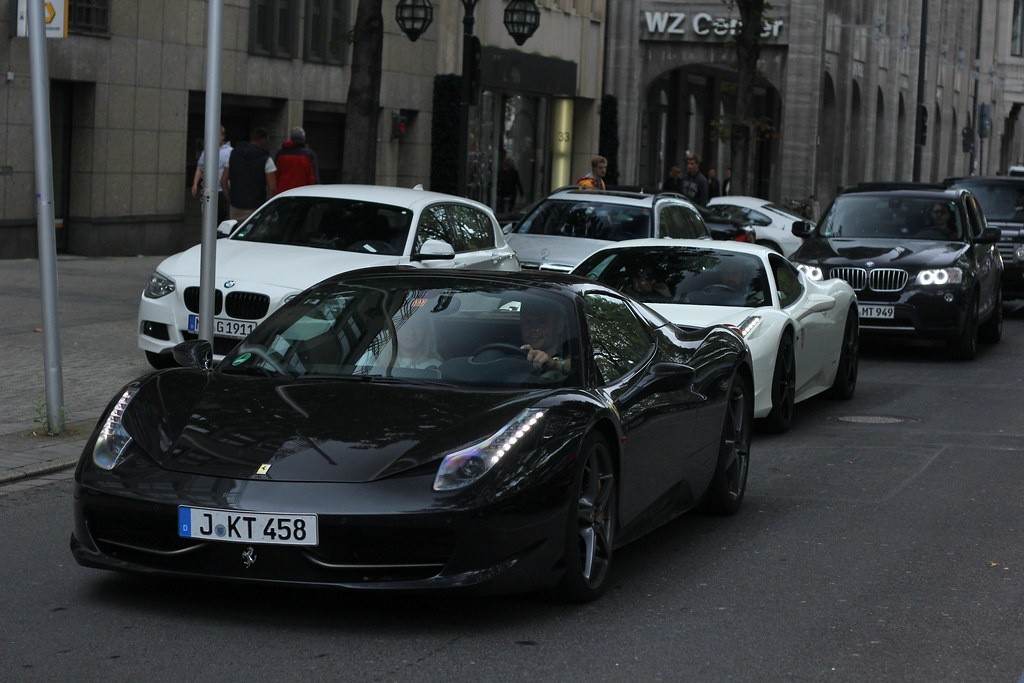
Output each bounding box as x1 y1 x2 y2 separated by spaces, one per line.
69 264 755 608
542 236 862 434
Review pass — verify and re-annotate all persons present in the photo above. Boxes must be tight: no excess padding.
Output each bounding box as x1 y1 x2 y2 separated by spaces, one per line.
379 302 444 371
664 153 733 205
192 123 319 239
922 202 958 241
630 265 674 300
502 159 523 213
517 295 572 381
577 156 606 191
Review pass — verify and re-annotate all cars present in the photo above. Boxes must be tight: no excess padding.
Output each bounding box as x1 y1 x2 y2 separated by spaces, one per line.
944 176 1024 298
697 196 818 259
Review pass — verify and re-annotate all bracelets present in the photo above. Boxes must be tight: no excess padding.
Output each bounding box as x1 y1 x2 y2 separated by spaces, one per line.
553 356 566 374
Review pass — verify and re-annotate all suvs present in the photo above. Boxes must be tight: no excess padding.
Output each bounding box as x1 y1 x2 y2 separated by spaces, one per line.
503 182 709 276
786 180 1006 360
134 182 518 370
